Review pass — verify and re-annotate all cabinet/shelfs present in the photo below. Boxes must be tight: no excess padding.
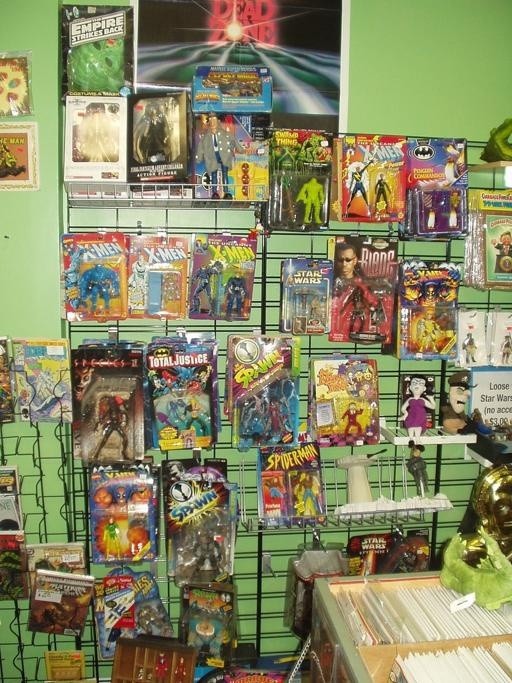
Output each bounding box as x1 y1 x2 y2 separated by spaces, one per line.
310 569 512 683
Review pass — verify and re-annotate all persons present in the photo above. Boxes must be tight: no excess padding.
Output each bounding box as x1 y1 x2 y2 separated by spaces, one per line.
225 270 249 320
400 376 437 438
445 370 471 434
192 530 225 578
409 444 432 495
144 108 174 164
331 244 387 340
197 114 245 198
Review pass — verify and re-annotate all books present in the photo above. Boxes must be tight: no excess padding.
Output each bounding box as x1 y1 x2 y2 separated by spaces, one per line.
0 464 96 636
1 336 73 424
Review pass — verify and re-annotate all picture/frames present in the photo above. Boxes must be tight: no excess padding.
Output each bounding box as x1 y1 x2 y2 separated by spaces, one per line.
468 367 512 431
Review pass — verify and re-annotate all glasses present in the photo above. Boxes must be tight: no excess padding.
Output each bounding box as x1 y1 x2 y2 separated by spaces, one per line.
337 258 354 262
